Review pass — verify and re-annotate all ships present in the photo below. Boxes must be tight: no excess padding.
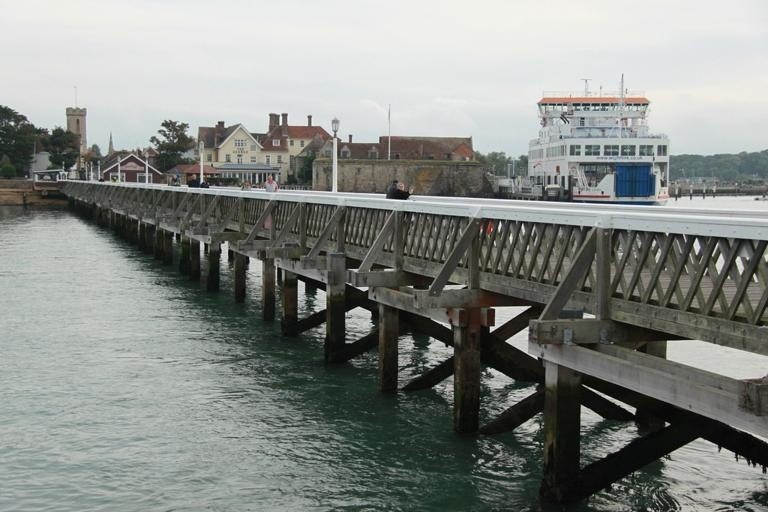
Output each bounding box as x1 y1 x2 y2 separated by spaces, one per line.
526 71 671 208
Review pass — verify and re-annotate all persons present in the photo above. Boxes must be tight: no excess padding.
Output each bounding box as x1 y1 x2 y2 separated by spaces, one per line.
99 176 105 182
168 173 181 187
240 181 252 191
111 177 119 183
187 174 200 188
200 178 209 188
390 182 416 200
24 173 28 180
386 180 399 199
255 175 278 192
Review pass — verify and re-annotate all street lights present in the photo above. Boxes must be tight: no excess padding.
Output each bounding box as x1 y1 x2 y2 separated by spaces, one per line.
198 139 204 184
83 161 101 181
144 152 149 184
117 156 121 182
331 118 340 192
